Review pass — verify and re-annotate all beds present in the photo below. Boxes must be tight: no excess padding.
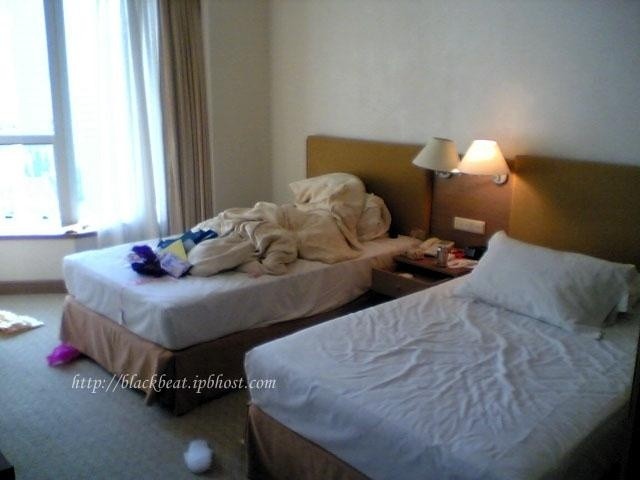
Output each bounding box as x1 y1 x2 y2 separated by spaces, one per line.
244 154 640 479
60 136 432 415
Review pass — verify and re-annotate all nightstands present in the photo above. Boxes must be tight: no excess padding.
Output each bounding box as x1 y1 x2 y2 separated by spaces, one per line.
371 246 482 297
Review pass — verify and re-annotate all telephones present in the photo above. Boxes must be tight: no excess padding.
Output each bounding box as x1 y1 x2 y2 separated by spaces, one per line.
416 237 455 256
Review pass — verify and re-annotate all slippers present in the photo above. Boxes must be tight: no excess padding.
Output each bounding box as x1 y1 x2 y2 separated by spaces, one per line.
184 440 214 474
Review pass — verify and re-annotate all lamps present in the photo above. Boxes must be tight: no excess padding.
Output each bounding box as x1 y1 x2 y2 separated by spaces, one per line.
411 137 462 179
458 139 510 185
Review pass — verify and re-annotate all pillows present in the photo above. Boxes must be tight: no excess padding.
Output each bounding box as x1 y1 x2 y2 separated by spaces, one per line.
455 230 637 340
356 193 392 242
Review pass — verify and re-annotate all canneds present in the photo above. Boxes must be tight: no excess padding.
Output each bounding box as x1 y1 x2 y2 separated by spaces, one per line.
436 245 448 267
